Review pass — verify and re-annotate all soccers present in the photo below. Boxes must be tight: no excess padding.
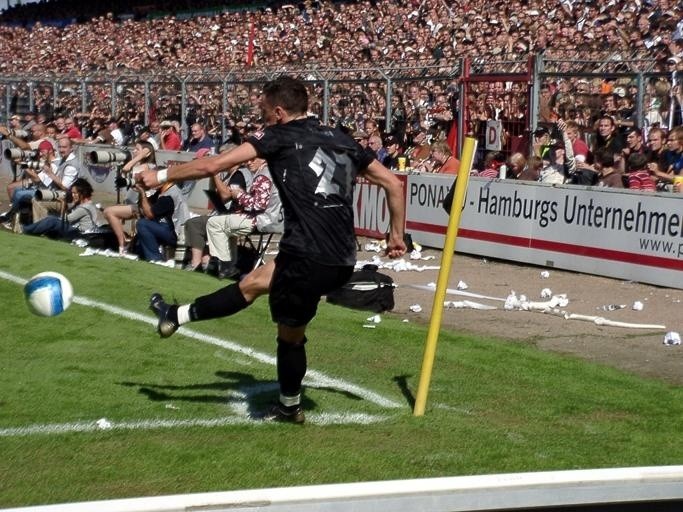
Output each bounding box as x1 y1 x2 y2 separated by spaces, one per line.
24 271 73 318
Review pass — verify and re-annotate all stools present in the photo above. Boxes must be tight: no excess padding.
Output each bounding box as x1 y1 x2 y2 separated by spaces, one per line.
235 230 283 274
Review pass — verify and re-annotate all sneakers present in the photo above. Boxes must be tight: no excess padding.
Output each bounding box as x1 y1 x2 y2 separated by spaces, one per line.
256 399 305 423
150 293 178 339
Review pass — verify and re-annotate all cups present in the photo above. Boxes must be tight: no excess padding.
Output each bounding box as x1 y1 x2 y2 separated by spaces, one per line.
397 156 406 172
672 175 682 185
229 183 240 190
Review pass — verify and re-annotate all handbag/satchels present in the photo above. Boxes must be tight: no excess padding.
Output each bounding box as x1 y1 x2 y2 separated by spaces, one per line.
327 265 395 313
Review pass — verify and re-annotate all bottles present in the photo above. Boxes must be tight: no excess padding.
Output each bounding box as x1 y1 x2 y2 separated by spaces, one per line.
498 163 507 180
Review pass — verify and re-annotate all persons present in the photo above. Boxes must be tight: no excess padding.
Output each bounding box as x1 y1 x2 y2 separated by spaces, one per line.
133 75 409 422
